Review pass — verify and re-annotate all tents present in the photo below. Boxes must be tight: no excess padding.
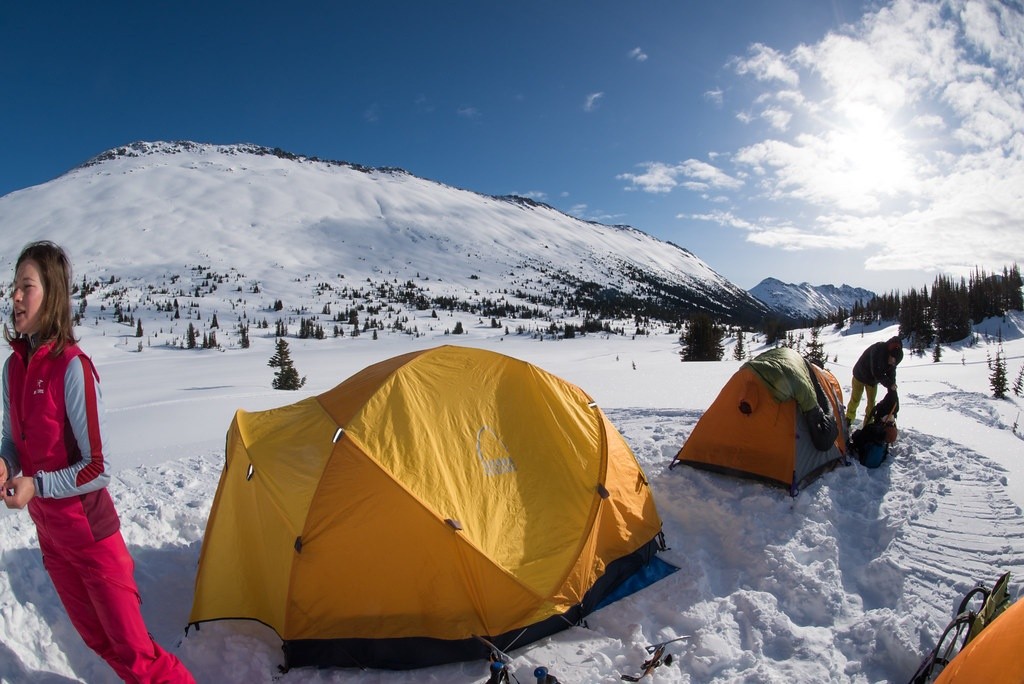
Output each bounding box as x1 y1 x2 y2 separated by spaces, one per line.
932 595 1023 684
668 345 853 500
186 343 681 684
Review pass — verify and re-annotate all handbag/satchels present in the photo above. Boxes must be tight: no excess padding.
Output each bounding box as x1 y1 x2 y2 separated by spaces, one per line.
872 390 899 443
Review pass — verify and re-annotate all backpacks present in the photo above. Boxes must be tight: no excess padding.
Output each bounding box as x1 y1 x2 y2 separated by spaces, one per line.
849 423 887 468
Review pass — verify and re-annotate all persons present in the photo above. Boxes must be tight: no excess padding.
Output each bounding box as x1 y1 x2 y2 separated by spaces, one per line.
0 236 196 683
845 336 903 429
